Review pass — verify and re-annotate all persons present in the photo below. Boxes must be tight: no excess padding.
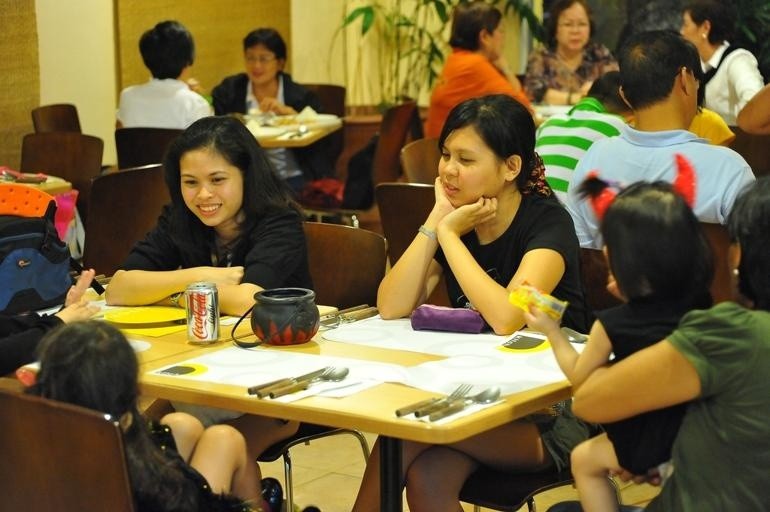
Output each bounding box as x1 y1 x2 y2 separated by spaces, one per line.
189 28 318 190
525 177 770 511
430 1 770 313
351 92 594 512
119 19 216 129
104 113 315 468
1 268 283 512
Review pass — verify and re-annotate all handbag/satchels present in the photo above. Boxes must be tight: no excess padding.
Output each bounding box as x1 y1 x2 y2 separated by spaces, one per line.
296 177 346 211
412 303 488 335
342 134 381 209
1 200 77 314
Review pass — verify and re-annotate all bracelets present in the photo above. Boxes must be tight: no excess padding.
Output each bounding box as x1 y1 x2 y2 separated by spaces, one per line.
418 221 437 242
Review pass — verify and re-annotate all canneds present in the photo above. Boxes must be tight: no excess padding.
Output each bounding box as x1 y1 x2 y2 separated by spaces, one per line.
184 282 220 346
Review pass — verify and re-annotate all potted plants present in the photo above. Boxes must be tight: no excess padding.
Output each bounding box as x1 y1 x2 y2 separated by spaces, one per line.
322 0 536 178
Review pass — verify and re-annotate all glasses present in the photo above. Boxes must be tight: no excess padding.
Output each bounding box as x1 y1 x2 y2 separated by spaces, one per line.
243 55 280 64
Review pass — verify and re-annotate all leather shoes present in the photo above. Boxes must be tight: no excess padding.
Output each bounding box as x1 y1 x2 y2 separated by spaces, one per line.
260 478 283 510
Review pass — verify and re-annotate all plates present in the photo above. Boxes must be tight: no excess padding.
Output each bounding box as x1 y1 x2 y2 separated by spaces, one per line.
104 306 187 325
267 112 339 129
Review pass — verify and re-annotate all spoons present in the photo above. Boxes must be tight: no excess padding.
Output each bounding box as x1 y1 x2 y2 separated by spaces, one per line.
429 385 501 421
269 367 349 399
288 126 309 139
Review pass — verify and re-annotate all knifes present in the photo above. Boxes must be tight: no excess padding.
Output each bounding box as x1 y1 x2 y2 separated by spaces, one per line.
319 304 370 326
247 367 326 395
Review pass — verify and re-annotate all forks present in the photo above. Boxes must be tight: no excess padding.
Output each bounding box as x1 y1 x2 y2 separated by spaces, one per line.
416 383 473 417
317 306 379 329
256 365 336 398
